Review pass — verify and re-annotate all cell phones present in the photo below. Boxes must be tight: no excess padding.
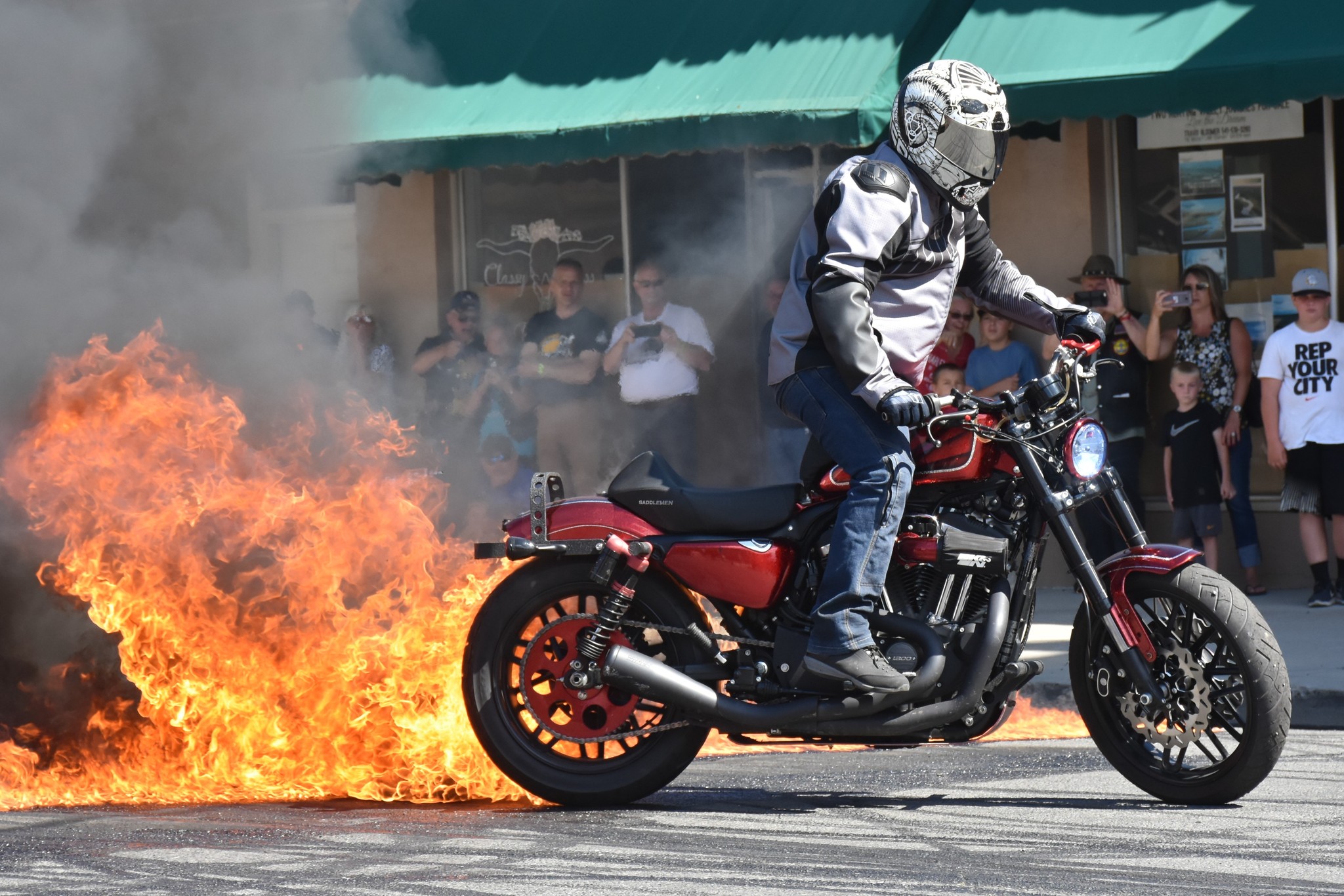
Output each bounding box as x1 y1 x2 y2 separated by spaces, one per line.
1073 289 1108 307
630 323 662 338
1162 291 1193 308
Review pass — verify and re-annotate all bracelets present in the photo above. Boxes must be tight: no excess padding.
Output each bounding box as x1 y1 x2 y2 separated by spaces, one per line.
1116 309 1132 323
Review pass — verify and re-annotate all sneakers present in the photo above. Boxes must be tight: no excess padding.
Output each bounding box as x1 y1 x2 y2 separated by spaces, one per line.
802 643 912 694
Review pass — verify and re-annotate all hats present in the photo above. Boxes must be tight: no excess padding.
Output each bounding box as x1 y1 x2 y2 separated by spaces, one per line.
450 291 481 313
1065 253 1131 287
1290 267 1330 297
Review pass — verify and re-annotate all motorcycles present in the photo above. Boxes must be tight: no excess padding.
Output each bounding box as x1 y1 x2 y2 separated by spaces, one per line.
458 292 1293 812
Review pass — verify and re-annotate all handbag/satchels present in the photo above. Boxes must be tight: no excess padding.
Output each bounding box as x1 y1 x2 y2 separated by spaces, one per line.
1241 373 1264 428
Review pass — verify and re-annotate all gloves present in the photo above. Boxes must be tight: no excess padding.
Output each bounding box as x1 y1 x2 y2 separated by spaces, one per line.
1058 307 1109 347
880 386 932 429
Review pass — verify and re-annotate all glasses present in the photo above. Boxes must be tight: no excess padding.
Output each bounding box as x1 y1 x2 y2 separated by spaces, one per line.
951 312 972 321
1181 283 1209 293
636 278 664 288
458 313 479 323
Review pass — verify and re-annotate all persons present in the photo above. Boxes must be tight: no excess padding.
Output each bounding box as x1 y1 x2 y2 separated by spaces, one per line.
604 260 716 485
1041 255 1147 591
1162 362 1236 573
914 290 975 396
465 319 536 498
336 316 393 380
761 277 812 485
766 58 1106 694
931 362 966 405
1257 268 1344 606
966 308 1042 402
412 291 485 507
516 258 613 502
1146 263 1266 595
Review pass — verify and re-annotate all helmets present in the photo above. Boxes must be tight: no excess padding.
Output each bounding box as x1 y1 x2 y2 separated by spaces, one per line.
889 59 1010 211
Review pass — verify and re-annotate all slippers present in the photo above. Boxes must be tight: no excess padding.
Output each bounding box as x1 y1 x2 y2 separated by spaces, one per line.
1246 583 1266 597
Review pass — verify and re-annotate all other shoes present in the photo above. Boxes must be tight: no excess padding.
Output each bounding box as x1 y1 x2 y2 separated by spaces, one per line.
1306 584 1344 607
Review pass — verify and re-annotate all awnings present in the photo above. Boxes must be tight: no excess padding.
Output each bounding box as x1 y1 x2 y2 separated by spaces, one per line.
321 0 933 184
929 0 1344 128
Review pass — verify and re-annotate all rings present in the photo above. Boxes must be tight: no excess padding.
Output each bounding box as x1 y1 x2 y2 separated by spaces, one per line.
1231 432 1235 437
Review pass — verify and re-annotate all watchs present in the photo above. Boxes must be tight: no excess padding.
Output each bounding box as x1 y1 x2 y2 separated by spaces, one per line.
537 363 544 376
1232 405 1242 412
